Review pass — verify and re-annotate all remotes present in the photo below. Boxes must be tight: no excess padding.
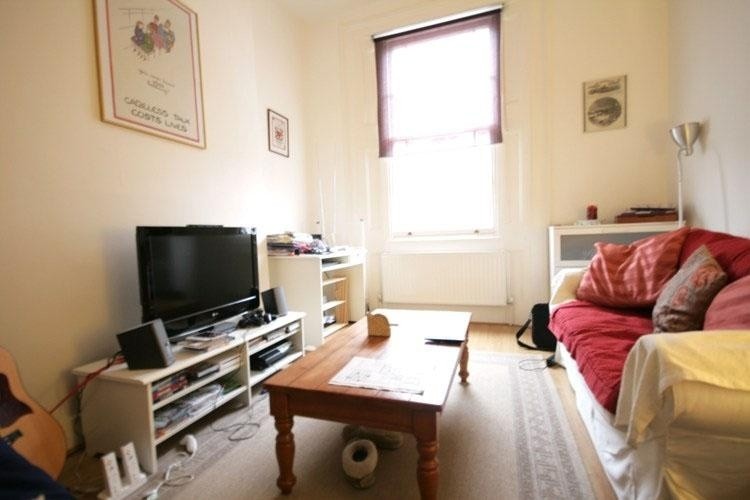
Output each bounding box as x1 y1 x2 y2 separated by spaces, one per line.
425 335 465 342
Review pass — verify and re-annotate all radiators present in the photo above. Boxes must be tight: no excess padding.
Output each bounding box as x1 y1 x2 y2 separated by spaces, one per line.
376 249 515 310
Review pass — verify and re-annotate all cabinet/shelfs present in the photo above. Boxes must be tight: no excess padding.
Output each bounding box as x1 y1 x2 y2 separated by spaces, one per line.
71 310 306 474
547 221 690 296
266 250 367 349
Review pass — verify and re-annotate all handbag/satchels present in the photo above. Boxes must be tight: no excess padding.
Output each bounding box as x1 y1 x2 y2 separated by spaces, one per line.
515 302 559 354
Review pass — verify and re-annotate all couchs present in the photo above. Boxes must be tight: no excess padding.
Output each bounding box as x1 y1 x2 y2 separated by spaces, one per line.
549 227 750 500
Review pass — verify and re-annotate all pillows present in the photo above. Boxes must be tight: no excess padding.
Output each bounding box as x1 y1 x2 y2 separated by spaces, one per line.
576 225 750 330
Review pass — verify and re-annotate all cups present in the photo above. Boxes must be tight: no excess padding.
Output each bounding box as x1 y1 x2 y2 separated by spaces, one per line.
587 203 598 219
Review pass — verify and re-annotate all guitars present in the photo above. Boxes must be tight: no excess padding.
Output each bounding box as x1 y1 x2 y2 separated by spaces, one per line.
2 350 70 496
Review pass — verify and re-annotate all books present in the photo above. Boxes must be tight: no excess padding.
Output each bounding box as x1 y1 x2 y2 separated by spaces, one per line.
152 363 226 433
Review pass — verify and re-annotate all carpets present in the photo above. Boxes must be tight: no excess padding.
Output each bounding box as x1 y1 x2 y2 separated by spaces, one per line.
88 350 598 500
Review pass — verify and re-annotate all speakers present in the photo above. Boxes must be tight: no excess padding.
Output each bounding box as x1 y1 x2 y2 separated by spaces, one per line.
261 287 288 314
116 319 175 370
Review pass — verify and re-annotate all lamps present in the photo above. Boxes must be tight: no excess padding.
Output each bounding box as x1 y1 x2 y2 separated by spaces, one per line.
667 120 701 227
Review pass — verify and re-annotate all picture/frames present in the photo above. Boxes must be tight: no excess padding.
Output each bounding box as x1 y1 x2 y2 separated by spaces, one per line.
92 1 206 152
267 108 289 158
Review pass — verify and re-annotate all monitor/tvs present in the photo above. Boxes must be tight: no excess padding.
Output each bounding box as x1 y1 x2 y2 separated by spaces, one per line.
136 224 259 342
